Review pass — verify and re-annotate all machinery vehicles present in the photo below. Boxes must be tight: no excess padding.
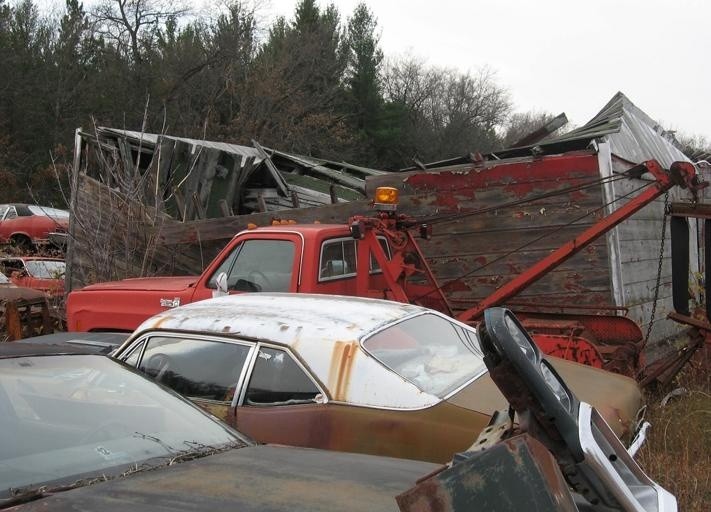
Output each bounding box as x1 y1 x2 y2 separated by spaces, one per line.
65 127 701 394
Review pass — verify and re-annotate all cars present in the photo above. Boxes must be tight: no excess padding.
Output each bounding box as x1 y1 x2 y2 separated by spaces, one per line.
3 288 657 490
0 253 68 295
0 272 55 345
0 344 483 511
0 201 68 257
